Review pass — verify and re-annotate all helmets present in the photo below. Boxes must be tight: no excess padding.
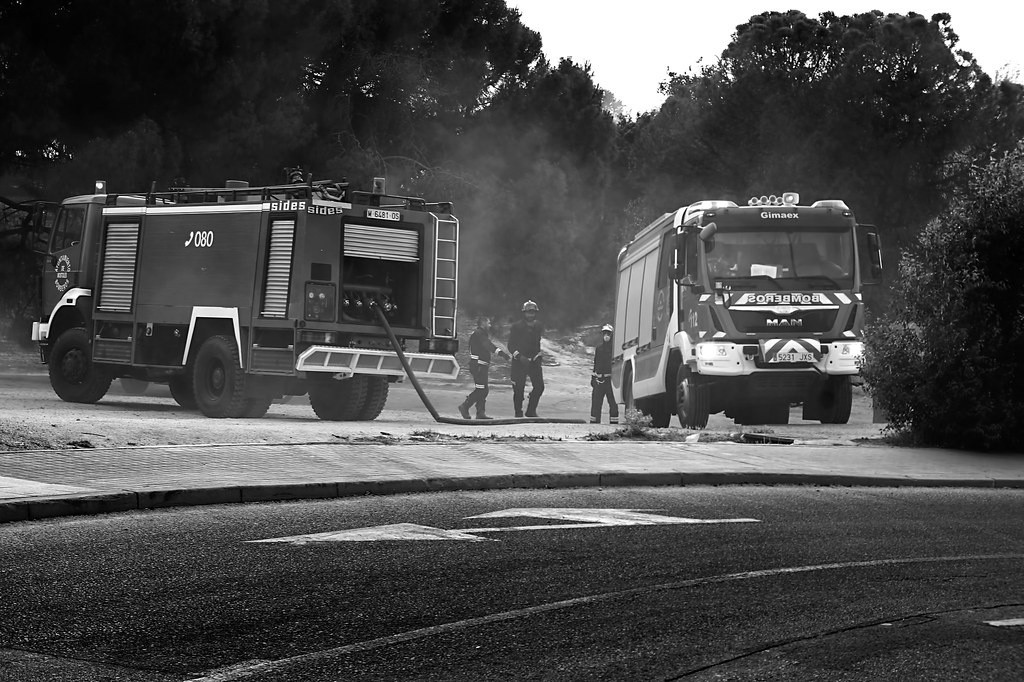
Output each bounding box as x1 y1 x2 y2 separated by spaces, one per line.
602 323 614 332
521 300 540 311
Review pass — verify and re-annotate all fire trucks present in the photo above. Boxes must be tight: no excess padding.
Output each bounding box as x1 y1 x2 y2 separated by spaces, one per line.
23 166 461 424
611 191 887 430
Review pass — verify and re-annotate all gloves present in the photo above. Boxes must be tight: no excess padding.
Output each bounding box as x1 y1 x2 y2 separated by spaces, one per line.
502 353 511 360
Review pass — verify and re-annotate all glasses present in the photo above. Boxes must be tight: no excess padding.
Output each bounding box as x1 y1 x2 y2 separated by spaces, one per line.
602 331 612 335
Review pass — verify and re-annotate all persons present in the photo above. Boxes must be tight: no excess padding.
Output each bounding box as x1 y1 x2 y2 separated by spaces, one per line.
458 315 511 420
588 323 621 424
507 300 545 417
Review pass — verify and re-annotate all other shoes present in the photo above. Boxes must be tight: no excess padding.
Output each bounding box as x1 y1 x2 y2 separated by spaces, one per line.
526 410 539 417
476 415 492 419
459 404 472 418
